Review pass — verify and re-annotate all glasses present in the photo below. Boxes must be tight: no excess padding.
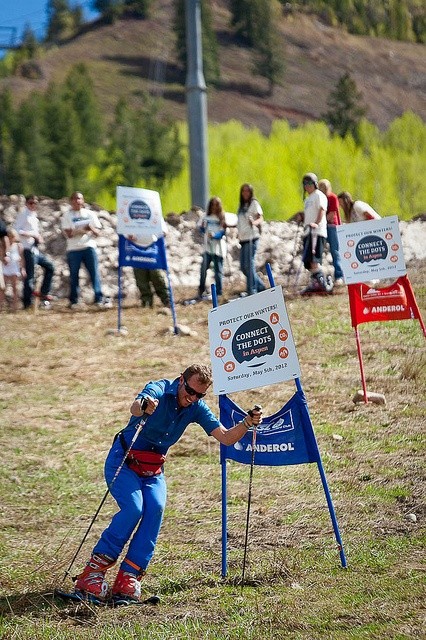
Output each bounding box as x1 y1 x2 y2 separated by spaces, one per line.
183 379 207 400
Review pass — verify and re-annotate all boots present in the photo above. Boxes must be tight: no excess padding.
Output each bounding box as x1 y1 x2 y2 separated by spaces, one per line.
75 553 116 601
111 569 142 601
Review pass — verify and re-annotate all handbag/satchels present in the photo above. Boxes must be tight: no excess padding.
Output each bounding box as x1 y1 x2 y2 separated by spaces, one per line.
125 449 167 477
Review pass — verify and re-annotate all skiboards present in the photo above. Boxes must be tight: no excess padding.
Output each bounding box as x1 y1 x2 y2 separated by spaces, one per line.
53 589 160 606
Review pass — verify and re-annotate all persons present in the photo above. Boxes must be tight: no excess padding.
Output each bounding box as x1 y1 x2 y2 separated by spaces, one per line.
3 228 27 311
318 179 345 287
227 183 267 296
196 197 226 301
0 217 10 314
15 194 55 310
133 217 170 308
75 364 263 601
61 191 110 309
302 172 334 293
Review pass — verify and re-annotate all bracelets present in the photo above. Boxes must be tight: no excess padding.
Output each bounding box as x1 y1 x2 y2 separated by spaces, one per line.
242 418 251 429
